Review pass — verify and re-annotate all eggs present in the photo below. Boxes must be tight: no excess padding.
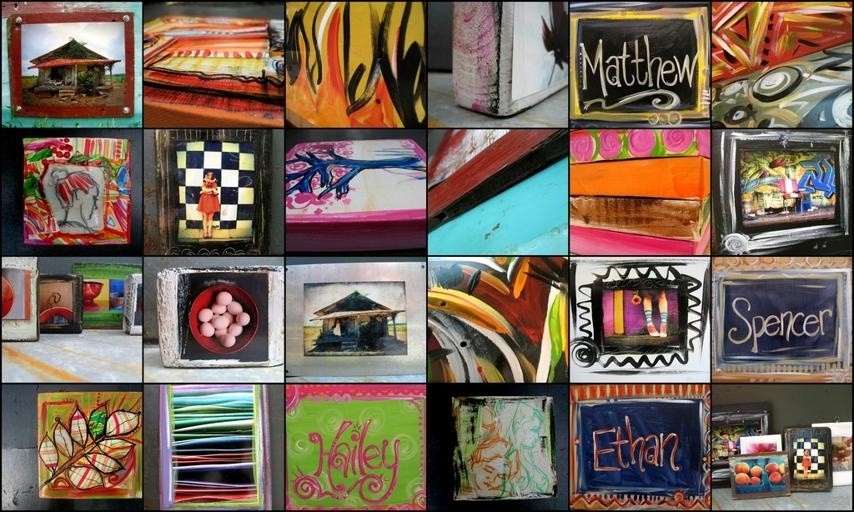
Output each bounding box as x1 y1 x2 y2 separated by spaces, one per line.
200 292 253 348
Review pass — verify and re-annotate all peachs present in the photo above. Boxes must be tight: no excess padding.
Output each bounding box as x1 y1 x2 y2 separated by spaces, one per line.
734 459 782 485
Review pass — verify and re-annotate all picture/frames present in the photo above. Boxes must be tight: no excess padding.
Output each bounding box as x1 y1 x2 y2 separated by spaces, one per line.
498 0 710 125
7 15 284 125
158 382 273 510
570 257 852 381
713 131 851 258
428 129 568 234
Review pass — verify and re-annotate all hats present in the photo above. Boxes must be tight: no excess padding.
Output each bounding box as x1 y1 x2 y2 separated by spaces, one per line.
203 172 216 181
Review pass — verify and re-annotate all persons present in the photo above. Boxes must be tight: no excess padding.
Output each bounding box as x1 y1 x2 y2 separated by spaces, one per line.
333 318 340 336
642 289 674 338
195 168 221 240
713 421 759 457
